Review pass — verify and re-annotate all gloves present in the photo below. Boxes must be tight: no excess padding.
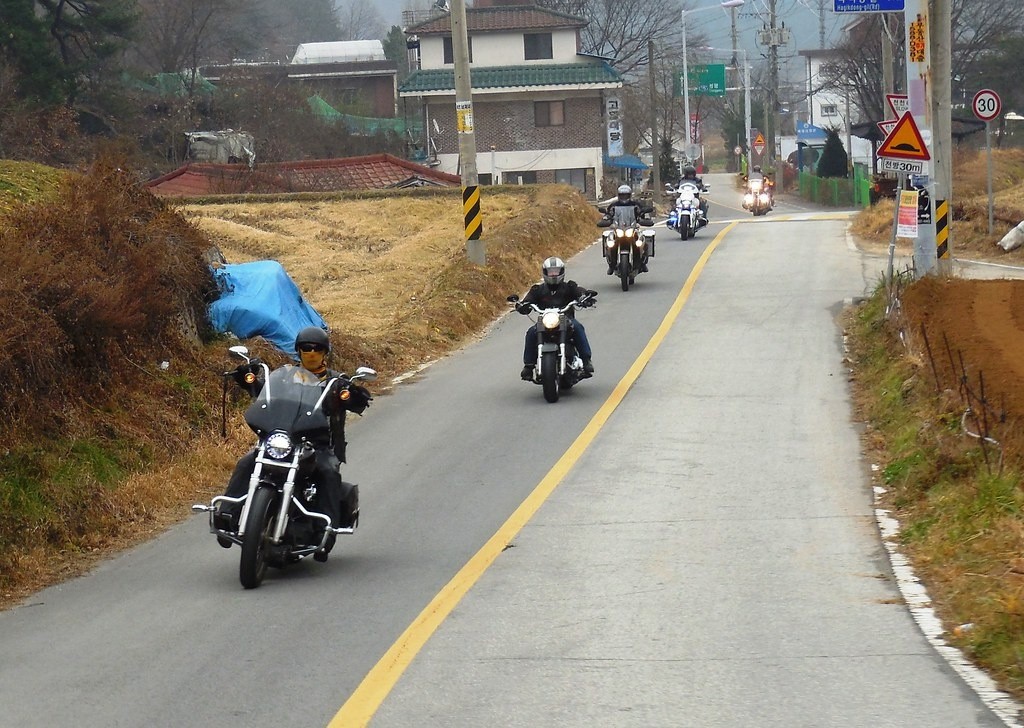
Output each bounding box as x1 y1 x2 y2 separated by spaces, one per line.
346 386 370 410
517 306 532 315
580 296 593 306
669 188 673 191
702 188 707 192
233 363 259 388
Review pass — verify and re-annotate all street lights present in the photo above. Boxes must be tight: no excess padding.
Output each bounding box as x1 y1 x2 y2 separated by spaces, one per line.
699 46 753 180
681 0 745 166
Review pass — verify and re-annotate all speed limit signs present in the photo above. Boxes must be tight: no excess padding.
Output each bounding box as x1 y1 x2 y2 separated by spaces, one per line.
971 89 1002 122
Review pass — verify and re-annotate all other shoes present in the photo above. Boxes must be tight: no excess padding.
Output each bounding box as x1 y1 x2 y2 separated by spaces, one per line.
581 358 594 373
521 365 535 379
208 513 235 548
607 266 615 275
643 265 649 272
702 219 708 226
314 533 336 563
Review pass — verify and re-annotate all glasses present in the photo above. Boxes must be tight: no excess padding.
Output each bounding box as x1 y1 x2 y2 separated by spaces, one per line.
299 345 325 352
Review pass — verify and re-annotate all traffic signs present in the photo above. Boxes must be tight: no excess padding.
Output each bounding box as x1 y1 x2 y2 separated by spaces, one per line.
672 63 726 98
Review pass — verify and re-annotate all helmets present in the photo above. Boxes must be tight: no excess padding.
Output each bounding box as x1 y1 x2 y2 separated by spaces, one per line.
617 185 632 204
684 166 696 175
542 257 565 287
754 165 761 173
295 327 329 352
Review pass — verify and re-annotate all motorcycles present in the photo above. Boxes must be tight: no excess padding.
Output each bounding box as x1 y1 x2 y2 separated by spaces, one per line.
191 346 378 589
738 171 778 216
596 207 656 292
506 290 599 403
664 178 711 241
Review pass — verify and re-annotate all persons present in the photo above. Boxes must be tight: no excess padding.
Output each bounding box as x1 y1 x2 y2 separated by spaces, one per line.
668 164 709 222
210 326 369 563
514 256 596 377
602 184 652 275
747 165 775 207
